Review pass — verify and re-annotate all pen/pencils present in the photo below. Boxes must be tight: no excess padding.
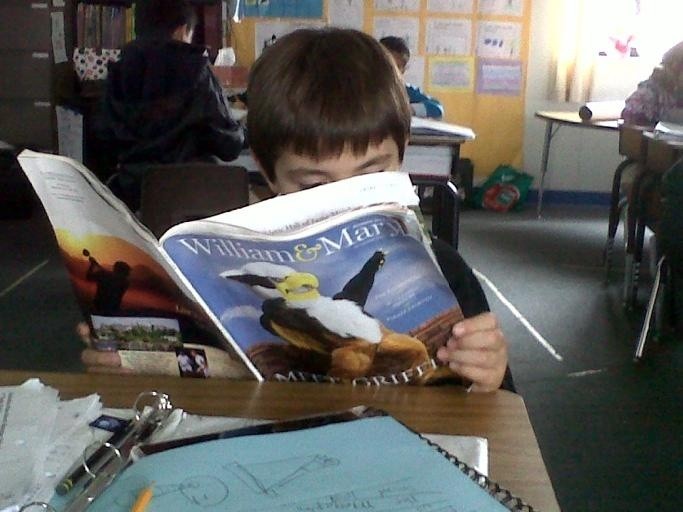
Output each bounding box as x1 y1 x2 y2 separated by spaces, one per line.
57 418 137 496
129 478 157 512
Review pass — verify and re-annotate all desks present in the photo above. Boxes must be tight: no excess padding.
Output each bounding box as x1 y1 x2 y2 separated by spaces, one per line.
534 110 683 363
0 369 562 512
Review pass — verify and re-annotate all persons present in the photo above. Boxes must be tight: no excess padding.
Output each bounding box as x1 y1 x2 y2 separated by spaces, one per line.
618 42 682 217
74 26 518 400
96 0 240 214
378 35 447 122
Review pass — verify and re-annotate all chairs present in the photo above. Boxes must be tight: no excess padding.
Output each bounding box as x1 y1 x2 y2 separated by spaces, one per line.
141 166 249 240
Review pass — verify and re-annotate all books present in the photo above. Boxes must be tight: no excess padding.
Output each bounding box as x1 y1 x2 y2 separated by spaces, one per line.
0 375 542 512
13 143 468 396
409 115 478 141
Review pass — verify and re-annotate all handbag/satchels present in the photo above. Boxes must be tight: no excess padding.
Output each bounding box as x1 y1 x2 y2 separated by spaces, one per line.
473 150 536 212
